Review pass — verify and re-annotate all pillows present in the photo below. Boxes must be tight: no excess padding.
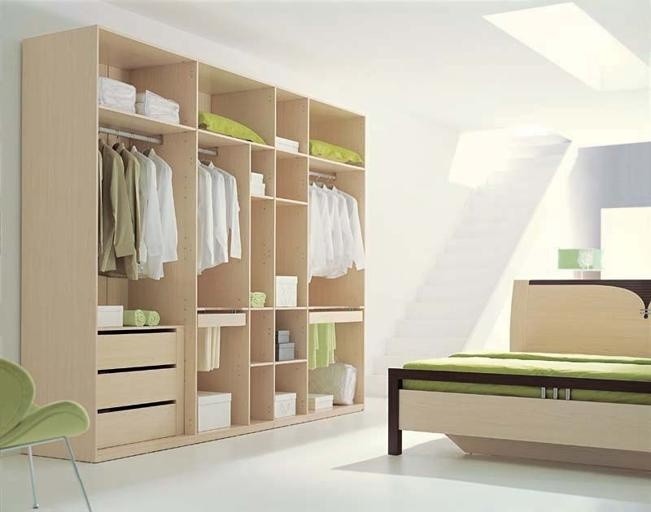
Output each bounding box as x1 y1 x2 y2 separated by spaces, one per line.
309 138 364 165
198 111 268 145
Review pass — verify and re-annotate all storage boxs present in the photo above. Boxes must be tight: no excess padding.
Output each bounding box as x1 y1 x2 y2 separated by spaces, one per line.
309 393 335 411
274 391 297 418
275 329 297 363
277 275 300 308
196 390 232 431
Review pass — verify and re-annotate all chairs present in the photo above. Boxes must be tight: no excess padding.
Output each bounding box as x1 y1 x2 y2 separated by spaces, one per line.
1 359 96 512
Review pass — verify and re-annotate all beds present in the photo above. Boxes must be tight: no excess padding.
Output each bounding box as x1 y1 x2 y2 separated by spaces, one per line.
388 281 651 457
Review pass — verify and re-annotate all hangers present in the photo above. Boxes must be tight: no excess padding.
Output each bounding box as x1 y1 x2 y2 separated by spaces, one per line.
97 122 175 173
308 172 358 206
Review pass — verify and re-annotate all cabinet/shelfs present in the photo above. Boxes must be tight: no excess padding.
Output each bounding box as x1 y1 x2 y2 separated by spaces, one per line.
18 22 369 466
